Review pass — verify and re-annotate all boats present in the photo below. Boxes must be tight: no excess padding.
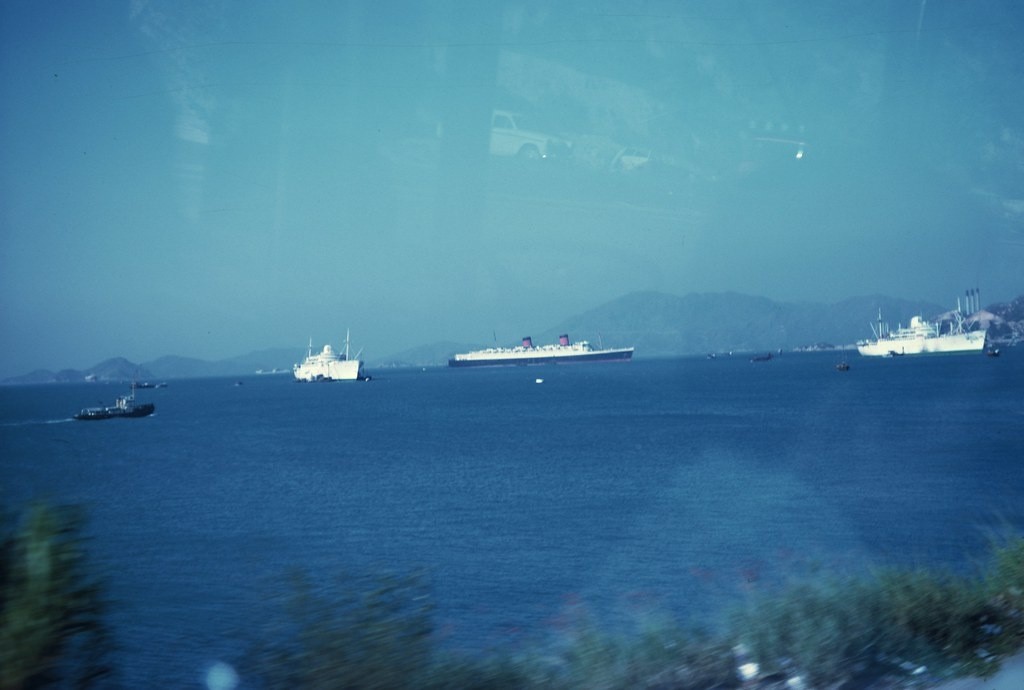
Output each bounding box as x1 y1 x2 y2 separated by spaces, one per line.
448 340 635 367
856 306 988 357
73 393 155 421
292 327 366 382
